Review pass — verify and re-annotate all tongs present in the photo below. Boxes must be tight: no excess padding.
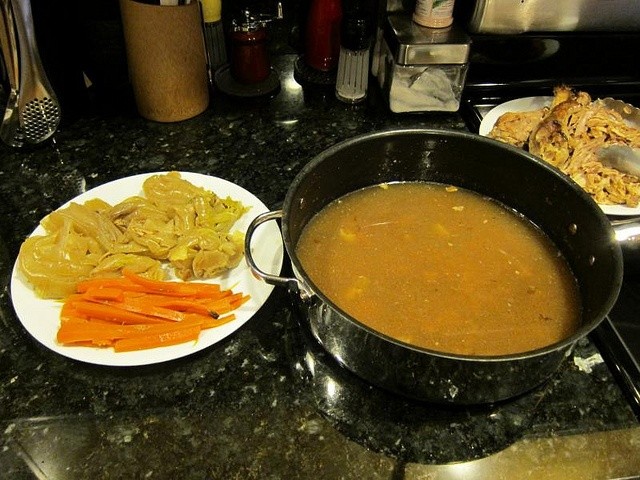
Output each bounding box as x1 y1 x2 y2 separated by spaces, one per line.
0 0 64 152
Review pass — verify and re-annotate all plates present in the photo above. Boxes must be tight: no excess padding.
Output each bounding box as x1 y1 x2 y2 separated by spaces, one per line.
477 95 640 216
10 169 285 367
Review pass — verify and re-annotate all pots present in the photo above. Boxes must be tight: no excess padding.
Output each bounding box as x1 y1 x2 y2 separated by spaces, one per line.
242 125 640 409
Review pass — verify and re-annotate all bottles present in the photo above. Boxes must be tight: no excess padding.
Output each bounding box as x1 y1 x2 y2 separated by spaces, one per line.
335 1 378 103
305 0 345 73
